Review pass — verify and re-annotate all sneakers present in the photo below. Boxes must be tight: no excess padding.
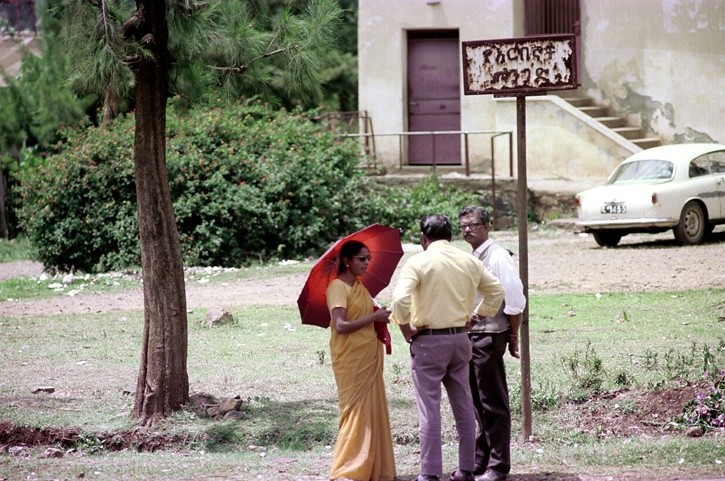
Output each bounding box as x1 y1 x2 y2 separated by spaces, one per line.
411 474 440 481
448 470 475 481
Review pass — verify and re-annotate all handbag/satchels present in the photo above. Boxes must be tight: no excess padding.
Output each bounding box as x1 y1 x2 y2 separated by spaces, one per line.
373 306 391 355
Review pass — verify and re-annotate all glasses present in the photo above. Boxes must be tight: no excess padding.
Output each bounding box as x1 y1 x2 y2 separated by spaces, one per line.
353 255 371 262
459 223 486 231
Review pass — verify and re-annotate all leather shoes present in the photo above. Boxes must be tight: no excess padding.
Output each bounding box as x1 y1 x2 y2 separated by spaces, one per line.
474 463 487 475
475 468 506 481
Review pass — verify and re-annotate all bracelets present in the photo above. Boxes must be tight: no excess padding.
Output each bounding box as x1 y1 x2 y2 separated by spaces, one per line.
509 334 518 338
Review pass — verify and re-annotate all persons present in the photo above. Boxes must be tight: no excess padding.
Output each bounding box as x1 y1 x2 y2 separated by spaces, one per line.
391 214 505 481
458 205 526 481
324 240 396 481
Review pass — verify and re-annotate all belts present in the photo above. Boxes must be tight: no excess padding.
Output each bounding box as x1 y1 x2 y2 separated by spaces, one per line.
418 326 465 336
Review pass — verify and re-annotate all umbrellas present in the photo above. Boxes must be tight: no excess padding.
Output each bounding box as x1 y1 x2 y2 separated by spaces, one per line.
297 222 405 329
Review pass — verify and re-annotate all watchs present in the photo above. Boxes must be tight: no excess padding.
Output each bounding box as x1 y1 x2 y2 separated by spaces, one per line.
471 313 481 323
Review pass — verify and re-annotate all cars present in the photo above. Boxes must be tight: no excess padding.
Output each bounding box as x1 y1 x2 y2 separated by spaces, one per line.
574 143 725 248
